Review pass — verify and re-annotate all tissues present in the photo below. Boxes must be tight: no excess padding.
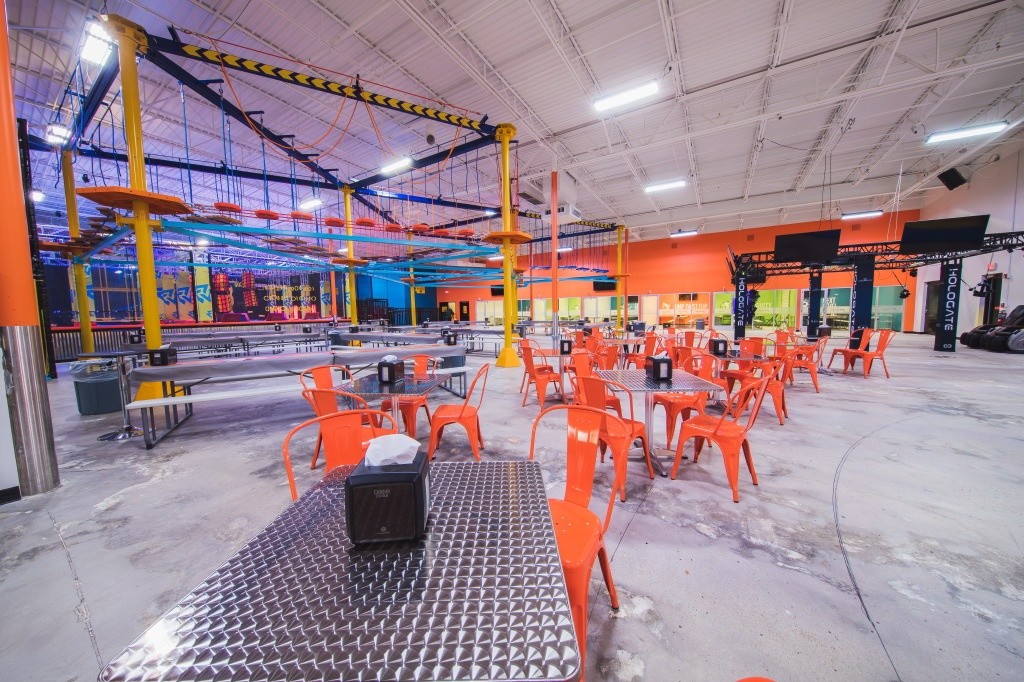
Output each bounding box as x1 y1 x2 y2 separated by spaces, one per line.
560 339 572 355
139 326 145 335
646 350 673 380
378 380 406 395
626 321 634 332
421 321 428 327
443 331 457 346
129 331 141 344
303 324 311 333
329 320 338 326
646 377 672 391
349 325 358 333
380 318 384 325
377 355 405 383
149 344 177 367
709 337 728 354
275 323 281 331
440 326 452 337
343 431 435 547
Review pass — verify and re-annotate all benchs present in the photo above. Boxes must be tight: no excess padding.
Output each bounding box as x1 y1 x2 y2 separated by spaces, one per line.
123 331 589 450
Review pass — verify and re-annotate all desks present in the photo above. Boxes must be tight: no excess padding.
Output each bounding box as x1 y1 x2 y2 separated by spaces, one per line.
700 349 769 409
95 460 580 682
77 349 164 442
330 373 449 433
124 318 589 381
595 369 725 477
660 333 706 346
597 338 642 370
765 340 810 356
531 348 588 401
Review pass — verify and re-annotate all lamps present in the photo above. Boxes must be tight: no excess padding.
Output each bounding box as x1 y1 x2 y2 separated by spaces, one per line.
593 82 698 237
841 209 884 219
926 121 1011 144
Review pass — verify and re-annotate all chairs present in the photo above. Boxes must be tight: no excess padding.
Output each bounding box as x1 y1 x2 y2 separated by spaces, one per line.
528 405 625 682
283 326 896 503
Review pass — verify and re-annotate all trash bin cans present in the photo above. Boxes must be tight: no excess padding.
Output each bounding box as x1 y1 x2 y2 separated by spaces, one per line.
635 321 645 337
329 330 349 354
71 359 130 415
696 318 704 330
